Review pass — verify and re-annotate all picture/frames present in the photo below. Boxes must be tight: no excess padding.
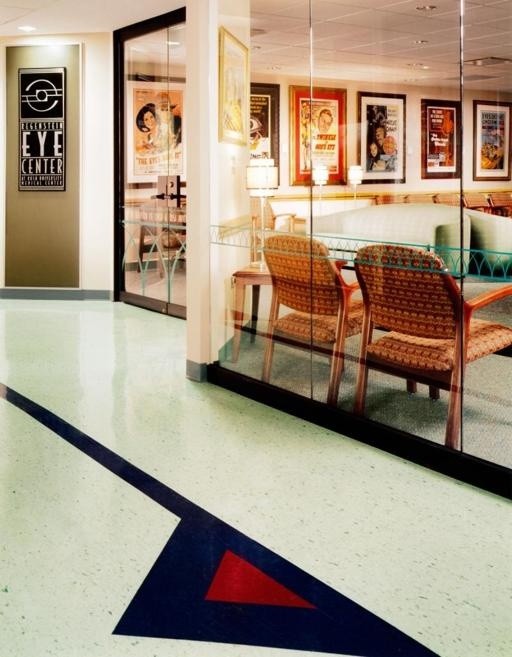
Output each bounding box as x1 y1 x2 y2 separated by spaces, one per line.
469 96 512 181
286 83 351 187
356 89 410 186
126 71 187 188
417 98 464 181
249 79 284 184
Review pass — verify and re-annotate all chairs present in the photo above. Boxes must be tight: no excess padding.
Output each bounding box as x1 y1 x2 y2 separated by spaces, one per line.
374 192 512 217
357 242 512 452
258 237 371 407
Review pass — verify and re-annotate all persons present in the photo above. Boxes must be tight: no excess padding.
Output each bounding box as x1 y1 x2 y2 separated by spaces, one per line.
443 109 454 167
143 111 159 142
379 135 399 173
370 127 387 171
317 108 333 134
368 142 382 170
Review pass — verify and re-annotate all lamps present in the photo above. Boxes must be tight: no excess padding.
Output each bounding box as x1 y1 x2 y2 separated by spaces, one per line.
346 164 367 200
310 161 330 198
244 156 282 238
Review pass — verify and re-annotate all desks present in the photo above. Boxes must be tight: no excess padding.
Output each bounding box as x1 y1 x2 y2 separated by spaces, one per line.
233 263 274 362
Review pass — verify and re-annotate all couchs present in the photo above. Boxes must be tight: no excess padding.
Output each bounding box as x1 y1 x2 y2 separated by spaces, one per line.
292 204 512 278
